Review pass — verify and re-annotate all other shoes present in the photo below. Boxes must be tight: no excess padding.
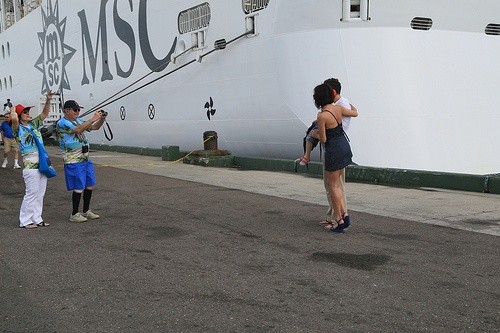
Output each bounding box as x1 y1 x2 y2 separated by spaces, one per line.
2 161 8 168
319 219 331 223
20 223 37 228
296 156 304 161
300 157 309 166
13 165 21 169
37 222 50 227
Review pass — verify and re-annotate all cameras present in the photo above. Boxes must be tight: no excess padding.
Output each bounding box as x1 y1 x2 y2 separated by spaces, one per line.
98 110 108 116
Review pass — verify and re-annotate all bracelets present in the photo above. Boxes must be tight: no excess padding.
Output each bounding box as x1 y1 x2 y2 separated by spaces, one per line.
46 102 50 104
11 112 16 115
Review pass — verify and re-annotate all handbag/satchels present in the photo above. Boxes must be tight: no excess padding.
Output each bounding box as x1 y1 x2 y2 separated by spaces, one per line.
38 145 56 177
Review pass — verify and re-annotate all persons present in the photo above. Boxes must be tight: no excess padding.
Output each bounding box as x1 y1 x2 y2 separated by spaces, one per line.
56 100 106 223
10 90 55 228
319 78 352 226
0 113 21 169
309 83 358 233
3 99 12 113
296 102 335 166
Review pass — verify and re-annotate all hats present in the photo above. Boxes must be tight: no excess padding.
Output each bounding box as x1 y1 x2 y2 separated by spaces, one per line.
64 100 84 110
16 104 35 116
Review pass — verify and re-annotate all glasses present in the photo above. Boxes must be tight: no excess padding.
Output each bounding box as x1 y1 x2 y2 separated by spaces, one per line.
68 108 81 112
22 110 30 114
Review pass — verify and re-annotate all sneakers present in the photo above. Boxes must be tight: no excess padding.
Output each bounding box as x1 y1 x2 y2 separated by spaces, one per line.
70 212 88 222
82 209 99 219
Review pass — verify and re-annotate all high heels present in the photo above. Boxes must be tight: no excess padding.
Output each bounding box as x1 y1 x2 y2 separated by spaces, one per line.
342 213 351 228
330 219 346 231
